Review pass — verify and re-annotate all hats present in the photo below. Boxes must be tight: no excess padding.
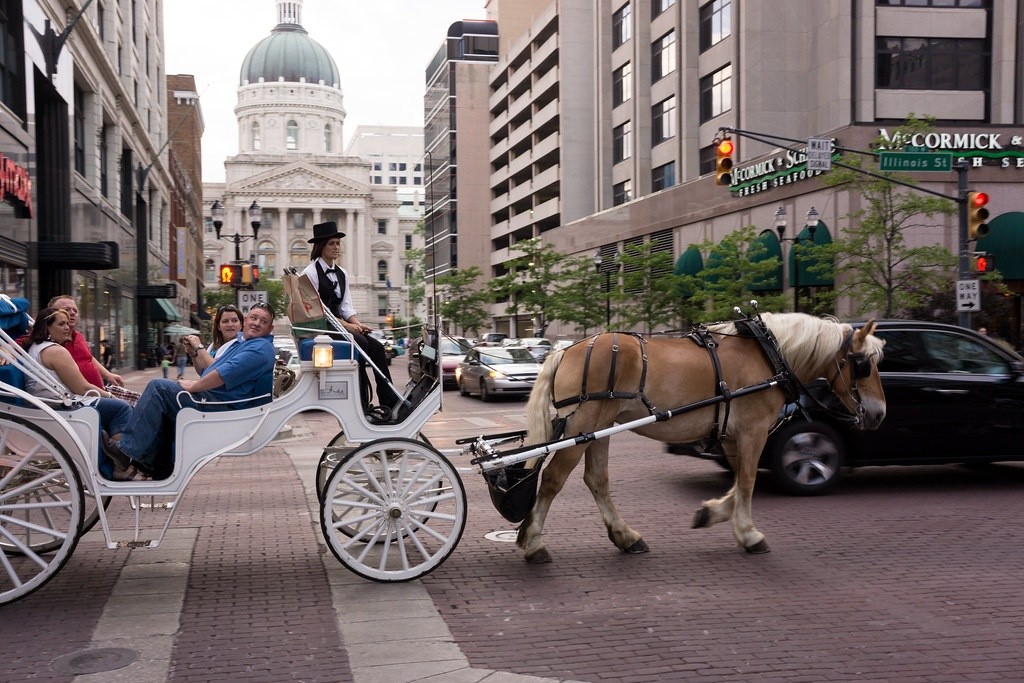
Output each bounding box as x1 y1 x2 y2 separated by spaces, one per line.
307 221 347 243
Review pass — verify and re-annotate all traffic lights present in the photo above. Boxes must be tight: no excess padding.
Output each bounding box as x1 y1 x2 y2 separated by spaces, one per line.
387 313 393 324
242 265 259 284
970 252 989 274
967 191 989 238
220 264 242 285
716 140 733 186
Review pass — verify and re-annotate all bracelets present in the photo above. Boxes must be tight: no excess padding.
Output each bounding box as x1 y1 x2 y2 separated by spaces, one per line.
108 392 112 398
191 352 197 358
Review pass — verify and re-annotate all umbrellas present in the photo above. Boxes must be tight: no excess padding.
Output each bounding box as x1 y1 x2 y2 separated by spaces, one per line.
163 325 203 336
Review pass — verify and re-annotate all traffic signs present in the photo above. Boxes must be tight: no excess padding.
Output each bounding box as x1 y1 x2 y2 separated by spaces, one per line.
956 280 981 312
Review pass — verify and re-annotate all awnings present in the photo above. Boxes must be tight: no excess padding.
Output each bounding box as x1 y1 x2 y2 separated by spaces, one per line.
147 299 175 321
788 219 835 286
672 244 704 297
163 298 183 322
974 212 1024 280
703 241 741 294
743 230 784 291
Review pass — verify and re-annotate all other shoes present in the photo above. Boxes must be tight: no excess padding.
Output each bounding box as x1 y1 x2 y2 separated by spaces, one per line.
177 374 184 379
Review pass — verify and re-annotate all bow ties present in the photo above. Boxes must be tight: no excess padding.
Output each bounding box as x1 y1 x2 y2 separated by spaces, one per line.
325 268 336 275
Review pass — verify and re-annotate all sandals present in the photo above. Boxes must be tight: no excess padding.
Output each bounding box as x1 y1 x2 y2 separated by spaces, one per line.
110 470 153 481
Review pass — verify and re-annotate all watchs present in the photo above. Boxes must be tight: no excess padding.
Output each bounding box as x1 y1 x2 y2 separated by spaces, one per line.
195 344 204 351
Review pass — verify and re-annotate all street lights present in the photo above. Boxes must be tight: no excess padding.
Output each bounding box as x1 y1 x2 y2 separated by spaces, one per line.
210 200 263 307
593 251 622 332
775 206 819 312
406 264 414 339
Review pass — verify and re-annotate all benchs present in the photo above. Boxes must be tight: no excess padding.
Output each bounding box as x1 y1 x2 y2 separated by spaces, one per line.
0 300 104 465
163 355 282 472
278 268 367 371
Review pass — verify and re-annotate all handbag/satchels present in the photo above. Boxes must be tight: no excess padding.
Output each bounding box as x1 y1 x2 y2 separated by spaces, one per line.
104 383 142 408
281 266 328 338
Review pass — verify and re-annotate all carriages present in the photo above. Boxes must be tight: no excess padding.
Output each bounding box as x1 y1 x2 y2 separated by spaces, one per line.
0 300 886 606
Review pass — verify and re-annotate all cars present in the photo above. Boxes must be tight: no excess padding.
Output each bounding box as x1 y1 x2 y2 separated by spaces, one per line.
367 329 405 365
667 318 1024 497
409 333 579 402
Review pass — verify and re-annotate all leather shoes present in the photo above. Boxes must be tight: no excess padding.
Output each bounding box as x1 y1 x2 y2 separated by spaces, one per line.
100 429 133 472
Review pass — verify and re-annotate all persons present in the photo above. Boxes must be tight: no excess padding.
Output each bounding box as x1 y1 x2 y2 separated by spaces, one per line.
99 339 112 370
182 303 244 376
0 293 126 391
100 302 275 475
158 337 193 379
299 220 411 410
24 309 155 482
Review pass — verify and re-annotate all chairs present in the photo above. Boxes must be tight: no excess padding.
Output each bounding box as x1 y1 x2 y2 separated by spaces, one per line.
884 339 919 371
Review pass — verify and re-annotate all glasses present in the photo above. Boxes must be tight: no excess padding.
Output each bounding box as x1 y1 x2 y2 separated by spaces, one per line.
219 304 239 311
251 302 274 318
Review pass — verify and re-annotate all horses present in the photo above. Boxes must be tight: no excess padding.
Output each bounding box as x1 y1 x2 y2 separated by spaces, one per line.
514 312 887 566
